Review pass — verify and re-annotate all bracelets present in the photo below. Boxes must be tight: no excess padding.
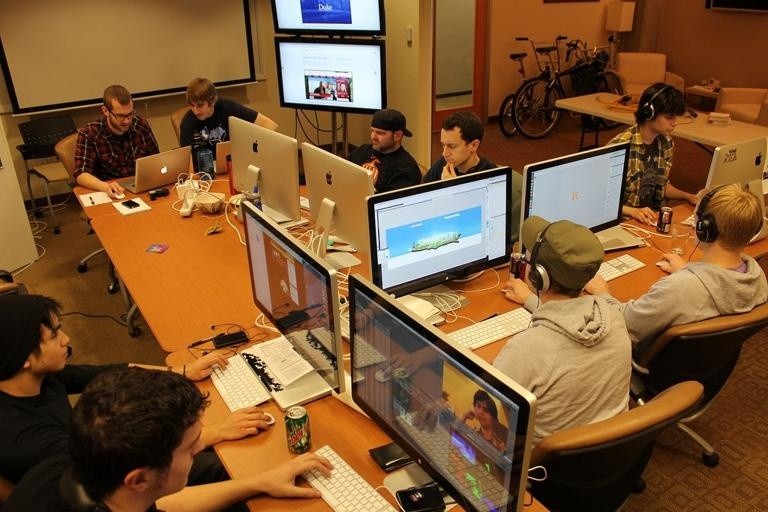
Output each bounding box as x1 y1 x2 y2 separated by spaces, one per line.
367 306 376 319
183 365 186 375
167 365 172 375
362 312 370 320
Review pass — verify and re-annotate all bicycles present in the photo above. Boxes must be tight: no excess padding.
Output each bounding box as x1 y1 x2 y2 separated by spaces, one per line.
494 33 569 136
512 36 628 135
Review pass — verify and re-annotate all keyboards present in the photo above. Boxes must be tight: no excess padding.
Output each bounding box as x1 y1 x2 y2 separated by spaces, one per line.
206 354 271 414
678 210 698 226
299 447 400 512
446 307 534 352
581 253 645 285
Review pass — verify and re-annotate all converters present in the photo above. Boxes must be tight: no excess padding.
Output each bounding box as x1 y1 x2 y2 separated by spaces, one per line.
185 179 199 191
176 183 192 197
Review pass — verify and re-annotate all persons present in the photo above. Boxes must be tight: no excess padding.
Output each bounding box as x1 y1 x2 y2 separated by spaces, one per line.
346 108 422 194
414 399 446 432
604 82 699 225
180 77 279 174
492 216 633 452
584 181 768 354
28 365 334 511
459 389 508 457
355 301 442 381
314 82 346 99
423 112 498 183
72 86 159 199
0 294 271 504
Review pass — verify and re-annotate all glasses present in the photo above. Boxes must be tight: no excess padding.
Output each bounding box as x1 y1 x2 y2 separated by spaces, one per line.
107 108 138 120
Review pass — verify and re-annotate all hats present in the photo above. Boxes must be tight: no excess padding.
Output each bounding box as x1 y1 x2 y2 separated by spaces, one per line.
520 216 602 290
370 109 412 137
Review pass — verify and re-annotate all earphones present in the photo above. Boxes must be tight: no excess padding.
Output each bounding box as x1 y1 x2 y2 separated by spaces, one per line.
211 325 216 329
203 351 209 355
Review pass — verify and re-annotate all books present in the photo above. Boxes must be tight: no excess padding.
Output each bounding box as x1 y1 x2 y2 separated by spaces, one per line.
709 112 731 124
395 294 446 326
112 197 152 216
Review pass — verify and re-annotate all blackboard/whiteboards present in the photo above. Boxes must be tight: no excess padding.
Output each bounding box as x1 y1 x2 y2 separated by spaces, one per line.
0 0 266 114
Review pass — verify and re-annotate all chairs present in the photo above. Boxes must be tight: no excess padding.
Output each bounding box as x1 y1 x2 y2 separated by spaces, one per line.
170 106 197 148
13 141 76 232
55 134 121 297
554 51 768 194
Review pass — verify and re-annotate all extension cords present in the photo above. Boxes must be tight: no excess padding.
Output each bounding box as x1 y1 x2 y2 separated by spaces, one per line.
181 194 194 216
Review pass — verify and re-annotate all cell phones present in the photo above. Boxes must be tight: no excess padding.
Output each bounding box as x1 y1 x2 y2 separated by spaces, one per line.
211 332 249 348
122 198 140 209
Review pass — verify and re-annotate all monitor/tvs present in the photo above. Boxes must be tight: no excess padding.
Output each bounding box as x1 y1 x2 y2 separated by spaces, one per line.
519 140 632 255
349 274 536 512
300 142 373 248
365 165 515 300
271 35 387 117
269 0 387 41
229 117 301 223
240 200 346 395
690 136 768 247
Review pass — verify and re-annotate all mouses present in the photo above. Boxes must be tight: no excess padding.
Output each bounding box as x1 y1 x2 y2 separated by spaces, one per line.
645 216 658 227
114 192 127 200
499 286 515 298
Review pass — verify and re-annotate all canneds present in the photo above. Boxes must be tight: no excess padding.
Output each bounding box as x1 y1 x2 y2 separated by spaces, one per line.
657 206 673 235
509 253 527 282
285 405 310 455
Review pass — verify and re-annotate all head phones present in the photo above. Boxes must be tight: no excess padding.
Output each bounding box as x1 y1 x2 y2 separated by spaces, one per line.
694 183 733 244
637 83 670 122
527 222 558 295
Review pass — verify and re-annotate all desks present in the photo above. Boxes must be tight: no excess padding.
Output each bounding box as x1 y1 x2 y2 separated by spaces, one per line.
0 157 768 512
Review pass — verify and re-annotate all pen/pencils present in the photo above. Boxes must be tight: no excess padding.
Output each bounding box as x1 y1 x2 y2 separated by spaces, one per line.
89 196 95 204
687 109 697 118
478 312 499 323
187 332 225 348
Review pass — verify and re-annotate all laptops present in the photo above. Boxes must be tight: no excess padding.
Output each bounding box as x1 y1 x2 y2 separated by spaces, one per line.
117 144 193 195
213 141 230 174
